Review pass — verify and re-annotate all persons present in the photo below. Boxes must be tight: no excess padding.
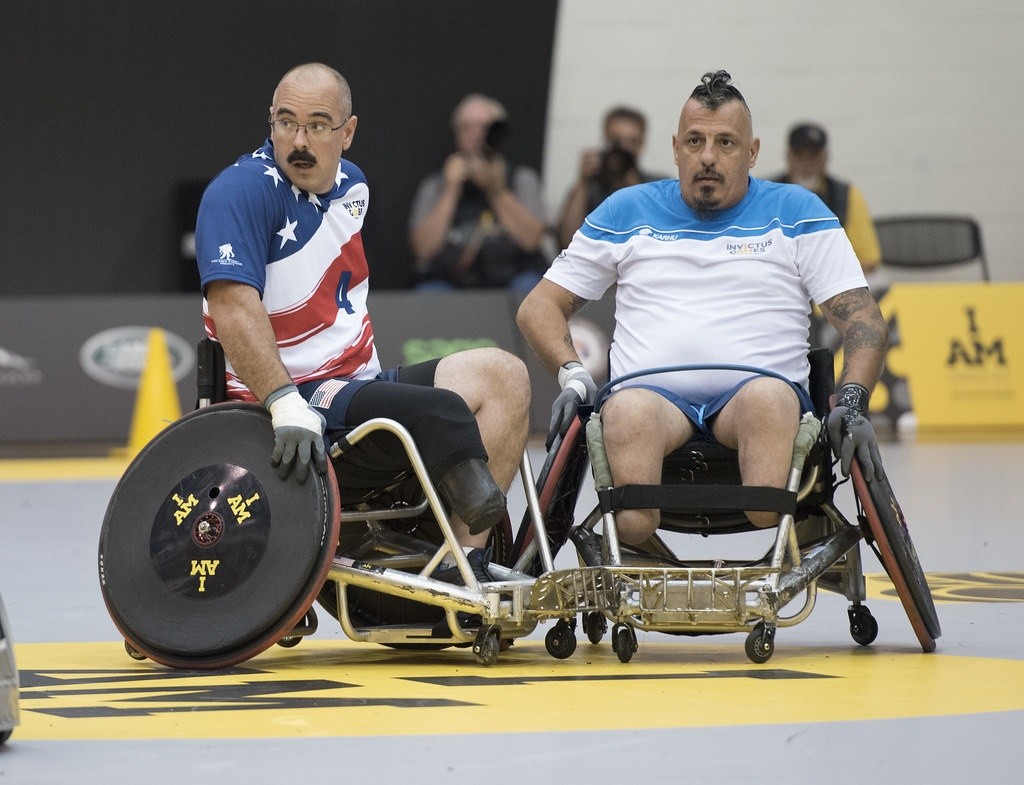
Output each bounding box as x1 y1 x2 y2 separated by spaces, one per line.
408 95 545 291
516 69 889 545
766 124 882 270
195 62 532 627
559 106 671 251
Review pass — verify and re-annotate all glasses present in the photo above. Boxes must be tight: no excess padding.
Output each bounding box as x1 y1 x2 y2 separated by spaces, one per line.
267 113 347 139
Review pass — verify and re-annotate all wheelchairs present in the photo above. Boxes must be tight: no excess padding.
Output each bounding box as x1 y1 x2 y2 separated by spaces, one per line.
97 337 620 675
495 347 943 664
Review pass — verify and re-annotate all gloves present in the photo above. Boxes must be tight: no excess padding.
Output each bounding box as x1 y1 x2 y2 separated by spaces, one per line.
828 383 883 484
545 362 598 453
264 383 327 484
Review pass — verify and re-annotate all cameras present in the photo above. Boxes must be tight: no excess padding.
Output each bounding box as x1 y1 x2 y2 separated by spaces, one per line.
599 144 635 176
480 120 512 160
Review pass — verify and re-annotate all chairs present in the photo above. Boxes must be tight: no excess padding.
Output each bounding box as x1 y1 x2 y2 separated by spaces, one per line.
875 216 988 281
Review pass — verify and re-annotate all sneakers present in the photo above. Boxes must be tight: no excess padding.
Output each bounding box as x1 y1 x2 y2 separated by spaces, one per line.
433 547 515 604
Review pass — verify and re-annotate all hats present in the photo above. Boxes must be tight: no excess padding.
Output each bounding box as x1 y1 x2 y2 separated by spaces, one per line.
790 125 826 150
